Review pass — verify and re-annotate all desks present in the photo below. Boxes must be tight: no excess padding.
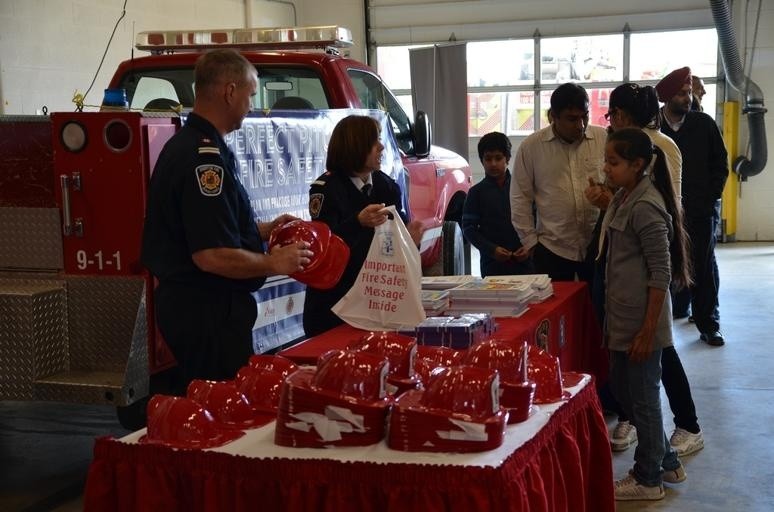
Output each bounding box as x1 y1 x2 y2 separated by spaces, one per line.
278 276 612 380
81 365 615 512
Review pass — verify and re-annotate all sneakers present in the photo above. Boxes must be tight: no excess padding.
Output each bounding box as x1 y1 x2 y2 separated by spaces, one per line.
669 426 704 457
659 465 686 483
610 420 638 451
613 469 665 500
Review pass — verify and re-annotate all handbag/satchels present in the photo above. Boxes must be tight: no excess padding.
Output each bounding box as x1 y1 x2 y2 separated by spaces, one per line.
330 205 427 332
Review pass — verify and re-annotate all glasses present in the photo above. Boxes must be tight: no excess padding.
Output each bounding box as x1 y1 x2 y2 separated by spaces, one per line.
604 109 616 121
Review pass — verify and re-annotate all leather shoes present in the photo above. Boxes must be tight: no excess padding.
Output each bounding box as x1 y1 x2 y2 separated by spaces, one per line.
700 330 724 346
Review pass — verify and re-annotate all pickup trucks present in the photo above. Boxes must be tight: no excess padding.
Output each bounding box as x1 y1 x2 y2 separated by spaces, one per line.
0 23 476 433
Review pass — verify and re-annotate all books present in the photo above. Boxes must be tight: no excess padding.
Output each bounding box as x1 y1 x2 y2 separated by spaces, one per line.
422 274 556 318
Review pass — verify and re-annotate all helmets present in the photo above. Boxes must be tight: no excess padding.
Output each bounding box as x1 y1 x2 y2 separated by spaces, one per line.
139 332 570 456
268 220 351 291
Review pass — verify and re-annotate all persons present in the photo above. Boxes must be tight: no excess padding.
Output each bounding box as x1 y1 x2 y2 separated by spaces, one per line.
463 66 728 500
141 47 314 397
304 116 407 336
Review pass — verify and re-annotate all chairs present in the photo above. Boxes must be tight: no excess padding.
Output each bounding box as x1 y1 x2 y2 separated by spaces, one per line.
272 97 314 111
146 98 178 111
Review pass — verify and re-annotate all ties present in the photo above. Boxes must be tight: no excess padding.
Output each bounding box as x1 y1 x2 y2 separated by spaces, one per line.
361 184 371 198
